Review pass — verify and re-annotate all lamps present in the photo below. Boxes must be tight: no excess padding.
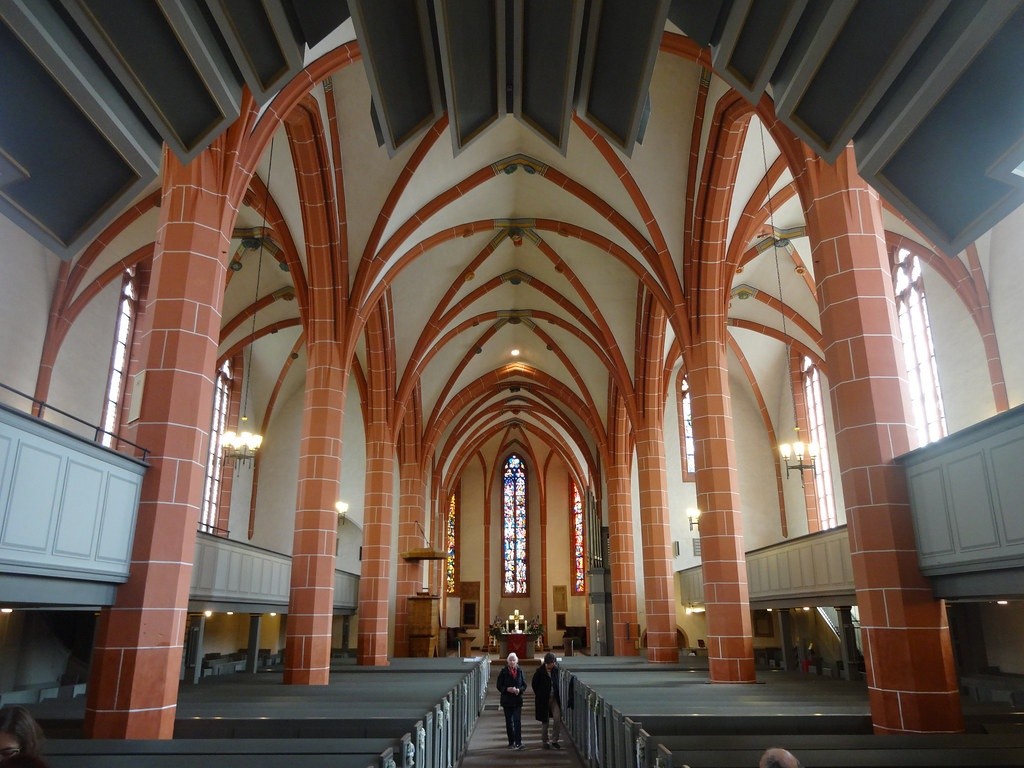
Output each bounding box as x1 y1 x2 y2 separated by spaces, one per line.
759 115 818 478
222 136 275 471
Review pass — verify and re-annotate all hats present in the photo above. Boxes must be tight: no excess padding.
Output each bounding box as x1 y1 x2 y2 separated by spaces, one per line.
544 653 556 662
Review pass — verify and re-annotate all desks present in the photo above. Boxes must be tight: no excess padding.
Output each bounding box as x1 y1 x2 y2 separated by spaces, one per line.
495 634 537 660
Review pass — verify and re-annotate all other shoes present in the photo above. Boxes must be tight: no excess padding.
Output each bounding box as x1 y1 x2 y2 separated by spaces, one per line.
509 744 513 748
515 743 521 749
552 743 560 748
544 743 550 749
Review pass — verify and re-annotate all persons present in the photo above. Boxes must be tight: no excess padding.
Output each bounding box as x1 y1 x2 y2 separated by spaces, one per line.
532 652 562 749
496 652 528 751
760 748 798 768
0 704 50 768
